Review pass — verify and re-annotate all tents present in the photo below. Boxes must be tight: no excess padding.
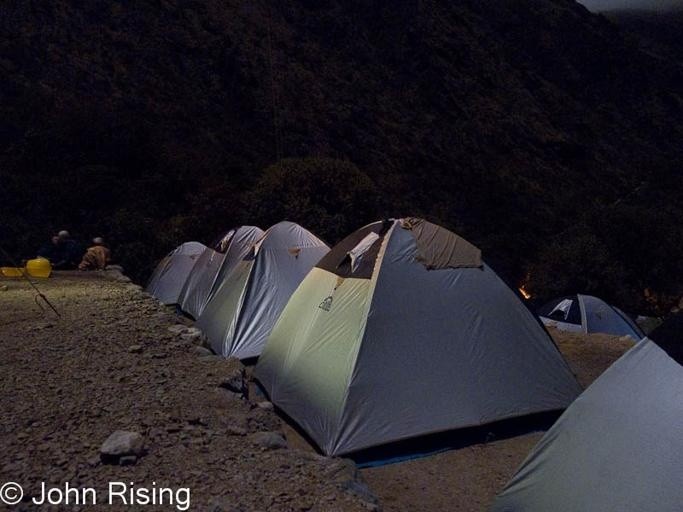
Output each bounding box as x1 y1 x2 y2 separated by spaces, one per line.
482 306 683 511
533 292 647 342
177 224 266 319
246 216 584 469
190 219 333 361
144 240 208 306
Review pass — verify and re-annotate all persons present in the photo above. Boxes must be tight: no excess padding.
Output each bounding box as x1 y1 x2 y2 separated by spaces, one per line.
77 236 112 271
49 228 77 268
